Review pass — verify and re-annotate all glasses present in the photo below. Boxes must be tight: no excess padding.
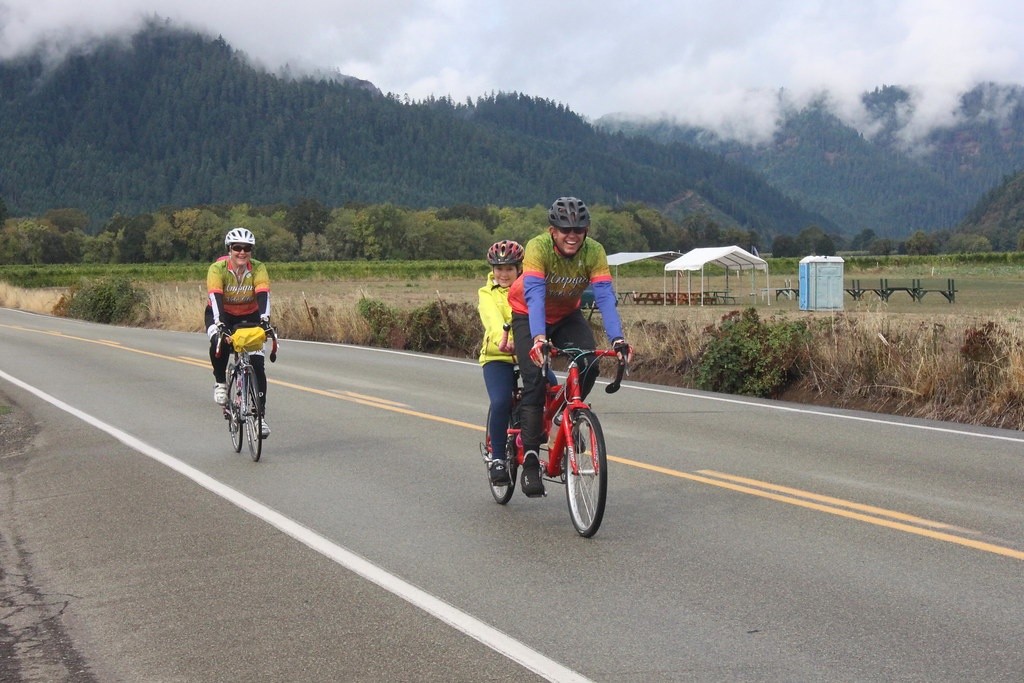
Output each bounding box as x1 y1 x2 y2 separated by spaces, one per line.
231 245 251 252
558 227 586 234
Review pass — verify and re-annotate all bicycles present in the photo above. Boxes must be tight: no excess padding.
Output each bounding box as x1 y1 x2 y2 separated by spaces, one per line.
214 316 279 462
477 322 634 539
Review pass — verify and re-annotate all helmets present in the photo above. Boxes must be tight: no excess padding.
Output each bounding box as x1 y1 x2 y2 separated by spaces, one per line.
224 228 255 246
548 197 591 228
487 240 525 265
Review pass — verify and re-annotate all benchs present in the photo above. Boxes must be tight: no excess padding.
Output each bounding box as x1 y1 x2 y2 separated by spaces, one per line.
612 277 960 304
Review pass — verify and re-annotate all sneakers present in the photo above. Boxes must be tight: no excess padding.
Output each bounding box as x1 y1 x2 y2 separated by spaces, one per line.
489 459 510 484
214 382 228 404
521 450 545 495
573 430 586 454
254 417 270 436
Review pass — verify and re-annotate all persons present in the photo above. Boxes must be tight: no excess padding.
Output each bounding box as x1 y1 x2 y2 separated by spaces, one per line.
204 227 271 439
475 239 560 486
506 195 636 497
